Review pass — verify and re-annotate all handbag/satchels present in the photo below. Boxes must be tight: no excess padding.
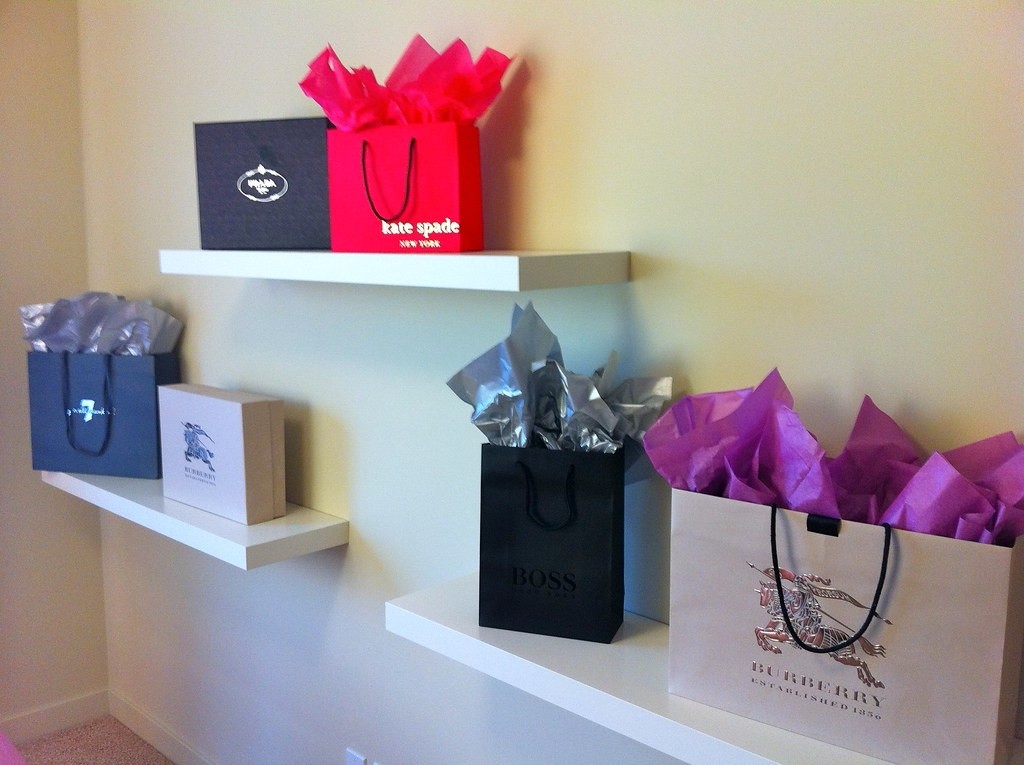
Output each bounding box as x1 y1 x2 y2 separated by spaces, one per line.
478 443 626 644
27 351 181 480
666 487 1024 765
327 126 484 254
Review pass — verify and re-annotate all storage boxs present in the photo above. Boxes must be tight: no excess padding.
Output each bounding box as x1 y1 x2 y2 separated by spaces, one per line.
195 116 337 248
158 381 289 524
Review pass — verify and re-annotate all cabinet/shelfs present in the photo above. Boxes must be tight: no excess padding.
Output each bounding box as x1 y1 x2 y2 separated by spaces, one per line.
36 249 888 765
